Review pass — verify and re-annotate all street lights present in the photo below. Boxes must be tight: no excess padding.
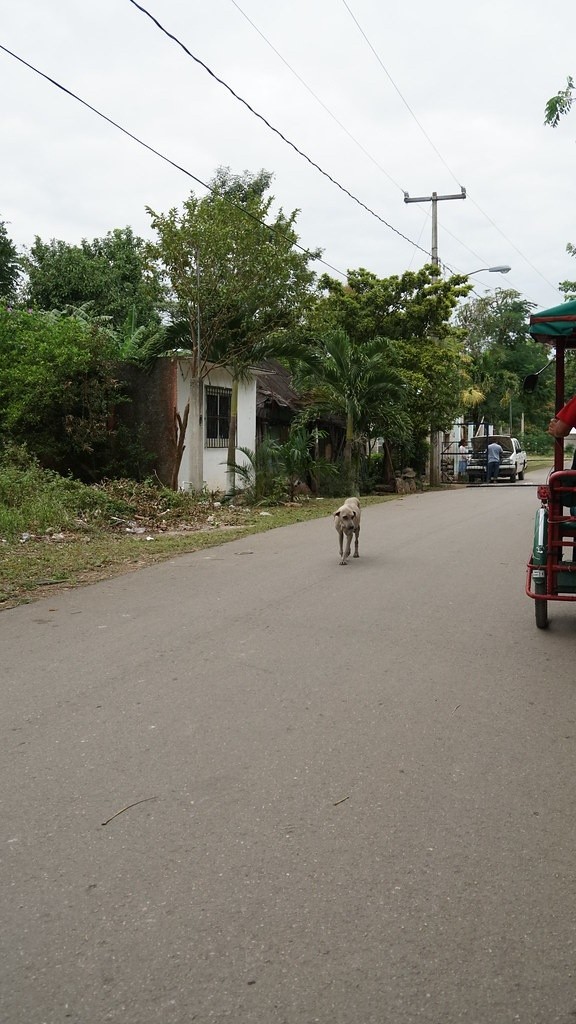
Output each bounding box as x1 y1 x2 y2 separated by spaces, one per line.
430 265 511 487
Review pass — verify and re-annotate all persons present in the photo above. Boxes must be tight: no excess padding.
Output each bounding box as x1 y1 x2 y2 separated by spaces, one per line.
548 394 576 470
484 439 503 483
458 439 468 481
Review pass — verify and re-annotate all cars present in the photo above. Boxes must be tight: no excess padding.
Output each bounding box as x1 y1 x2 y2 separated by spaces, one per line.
466 435 528 483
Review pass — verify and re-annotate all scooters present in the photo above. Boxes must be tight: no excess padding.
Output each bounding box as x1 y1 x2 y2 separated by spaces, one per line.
523 299 576 629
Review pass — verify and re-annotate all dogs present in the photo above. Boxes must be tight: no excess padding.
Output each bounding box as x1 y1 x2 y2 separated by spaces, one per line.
333 495 362 565
